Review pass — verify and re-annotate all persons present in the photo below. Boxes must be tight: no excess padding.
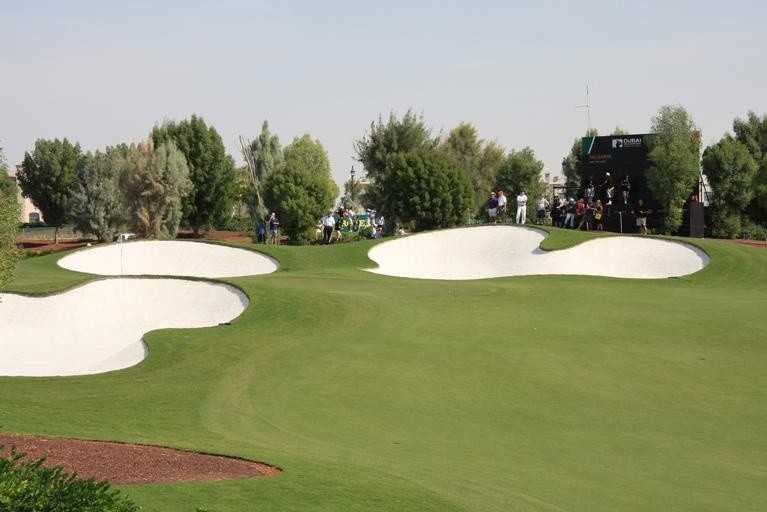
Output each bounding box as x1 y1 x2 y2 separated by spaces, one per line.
635 199 649 235
256 218 267 244
319 205 404 244
620 173 631 205
514 190 528 224
586 179 594 203
550 193 604 231
269 212 279 245
485 192 498 224
534 193 549 225
497 190 506 223
597 172 615 206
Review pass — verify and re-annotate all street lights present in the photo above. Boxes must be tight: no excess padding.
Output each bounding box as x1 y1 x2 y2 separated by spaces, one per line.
350 165 356 201
